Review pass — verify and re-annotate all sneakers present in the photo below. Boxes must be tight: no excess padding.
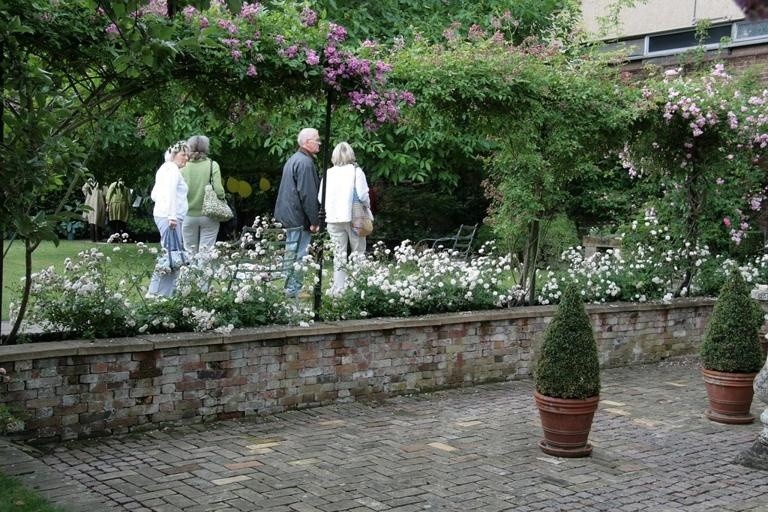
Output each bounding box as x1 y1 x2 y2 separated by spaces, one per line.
145 293 170 302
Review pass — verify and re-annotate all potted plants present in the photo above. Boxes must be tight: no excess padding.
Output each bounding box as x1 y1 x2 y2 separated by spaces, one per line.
533 282 601 457
700 266 765 424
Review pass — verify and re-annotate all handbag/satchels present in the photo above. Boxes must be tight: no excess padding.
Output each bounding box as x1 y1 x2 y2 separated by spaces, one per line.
161 225 191 274
202 158 234 222
350 164 374 237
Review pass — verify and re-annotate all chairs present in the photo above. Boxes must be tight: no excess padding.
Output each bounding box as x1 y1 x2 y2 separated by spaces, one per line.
208 225 304 292
415 224 479 260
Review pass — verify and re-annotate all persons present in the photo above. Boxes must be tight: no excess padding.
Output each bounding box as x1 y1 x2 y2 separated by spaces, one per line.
82 175 109 242
317 142 370 295
180 136 225 294
143 139 190 301
275 127 321 299
105 178 132 243
